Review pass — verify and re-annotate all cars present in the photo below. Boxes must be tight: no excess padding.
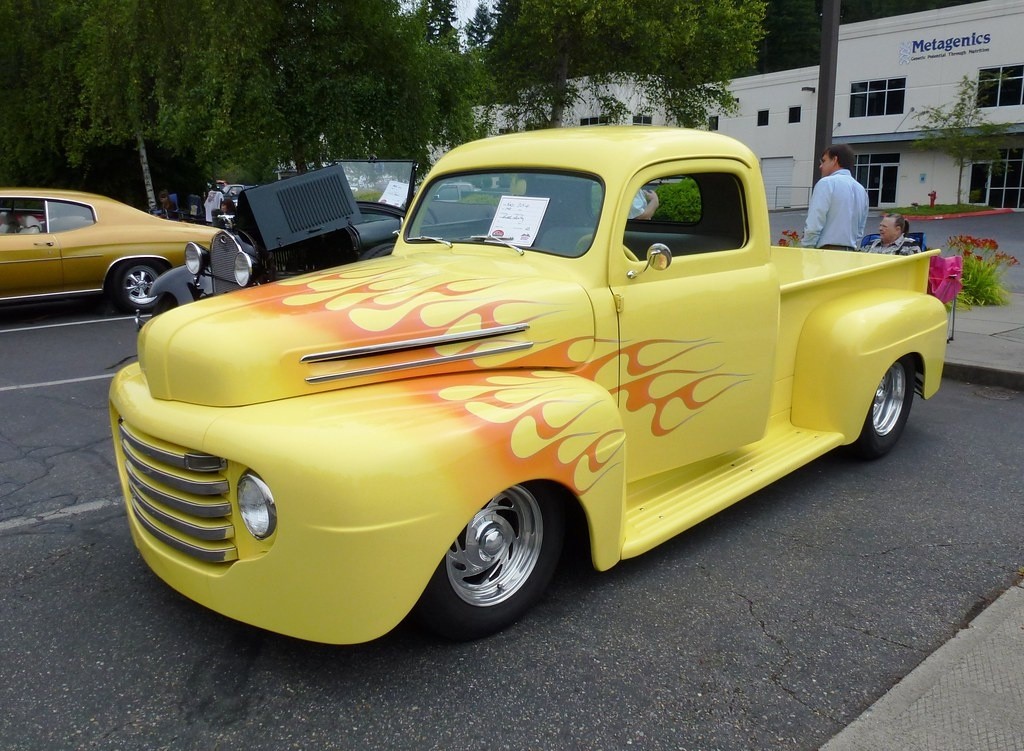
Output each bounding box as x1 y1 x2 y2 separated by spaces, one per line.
135 160 495 331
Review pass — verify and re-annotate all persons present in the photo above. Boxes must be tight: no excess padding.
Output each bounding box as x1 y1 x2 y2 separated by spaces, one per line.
859 213 921 256
157 189 179 221
800 143 869 252
628 187 660 220
218 199 236 229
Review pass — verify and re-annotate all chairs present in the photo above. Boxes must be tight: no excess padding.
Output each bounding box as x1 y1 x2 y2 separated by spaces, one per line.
0 212 15 233
148 193 207 225
860 233 963 343
17 214 43 234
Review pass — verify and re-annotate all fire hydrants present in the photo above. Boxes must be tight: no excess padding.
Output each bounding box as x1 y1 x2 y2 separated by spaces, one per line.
928 190 937 208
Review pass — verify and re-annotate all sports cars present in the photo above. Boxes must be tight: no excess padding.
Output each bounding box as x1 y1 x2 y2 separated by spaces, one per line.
0 188 222 314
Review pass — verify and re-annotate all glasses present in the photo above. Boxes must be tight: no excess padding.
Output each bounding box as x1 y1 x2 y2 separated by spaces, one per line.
820 156 834 164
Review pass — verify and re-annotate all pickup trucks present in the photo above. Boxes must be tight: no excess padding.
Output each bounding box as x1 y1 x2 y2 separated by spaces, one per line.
108 126 948 645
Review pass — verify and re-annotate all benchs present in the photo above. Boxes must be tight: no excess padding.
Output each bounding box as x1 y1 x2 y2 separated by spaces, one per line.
539 226 736 262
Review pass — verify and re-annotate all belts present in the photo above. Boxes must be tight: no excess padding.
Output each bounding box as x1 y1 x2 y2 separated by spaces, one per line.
821 244 855 251
